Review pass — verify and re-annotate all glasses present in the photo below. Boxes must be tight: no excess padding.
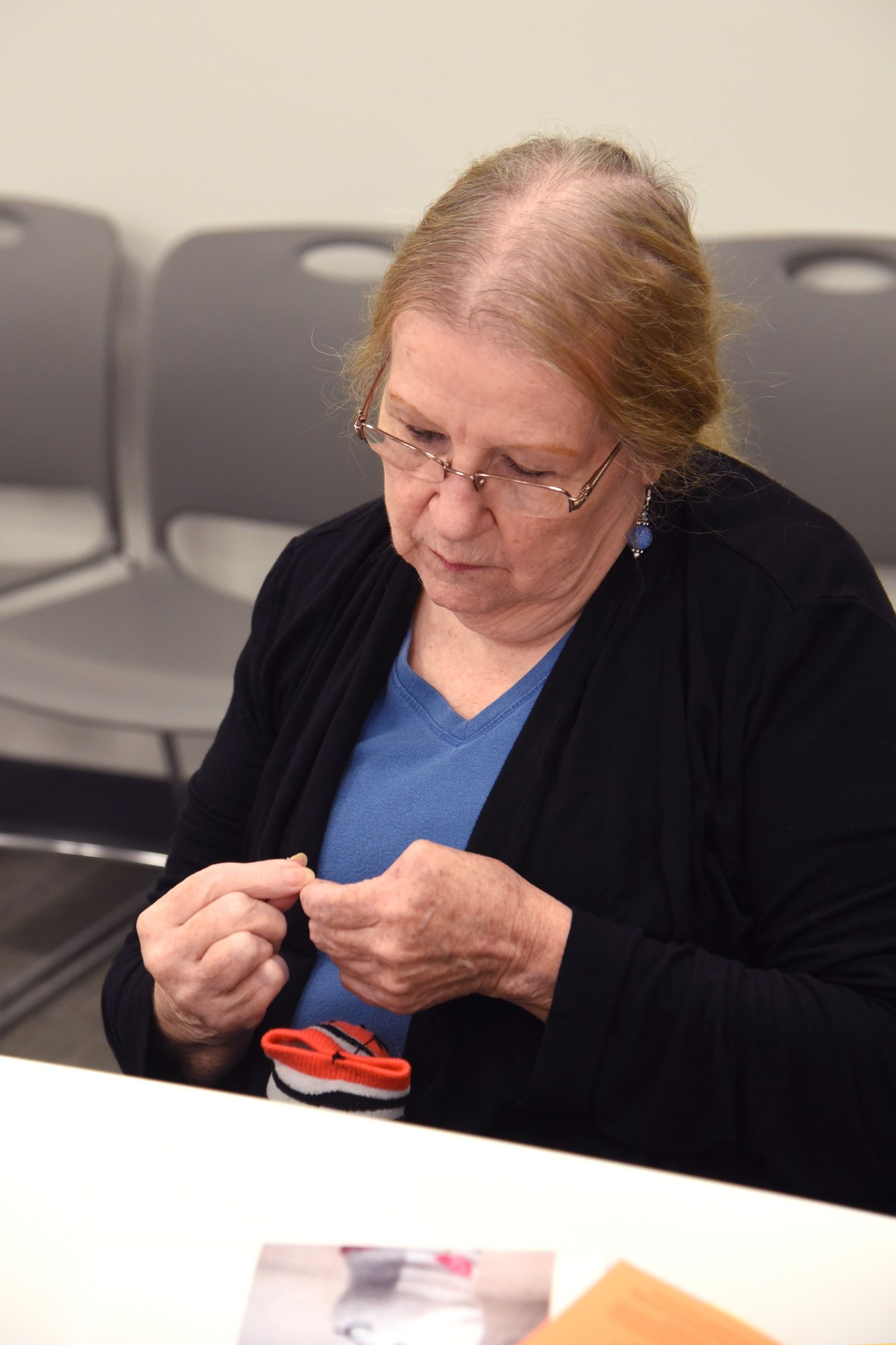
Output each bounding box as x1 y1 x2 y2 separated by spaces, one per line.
353 348 629 518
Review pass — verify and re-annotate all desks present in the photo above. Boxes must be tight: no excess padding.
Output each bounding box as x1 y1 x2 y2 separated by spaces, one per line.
1 1053 895 1344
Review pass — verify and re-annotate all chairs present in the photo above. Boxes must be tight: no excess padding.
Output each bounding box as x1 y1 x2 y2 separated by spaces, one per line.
0 227 410 1034
688 234 895 569
0 196 177 1001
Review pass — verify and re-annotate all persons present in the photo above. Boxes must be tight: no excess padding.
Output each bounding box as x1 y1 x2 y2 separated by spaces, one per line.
100 136 896 1222
